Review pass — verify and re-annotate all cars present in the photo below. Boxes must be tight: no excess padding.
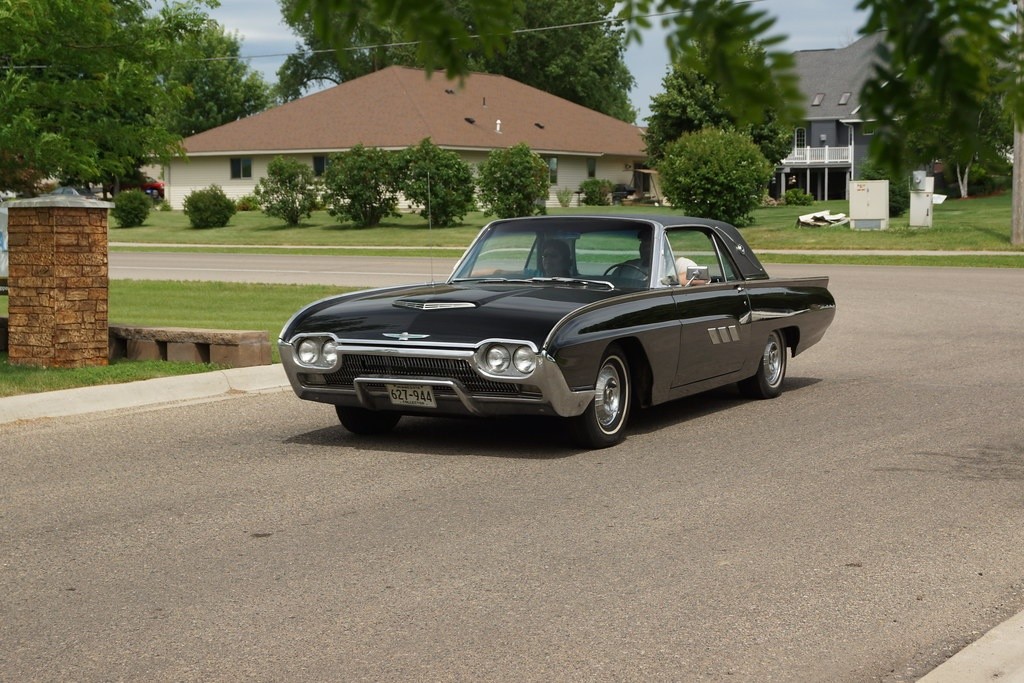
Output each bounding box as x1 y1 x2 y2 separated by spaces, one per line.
277 214 837 443
611 183 637 200
110 175 165 201
38 186 103 200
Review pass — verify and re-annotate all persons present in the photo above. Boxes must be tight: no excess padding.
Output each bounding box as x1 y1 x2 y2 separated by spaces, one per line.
612 230 711 286
471 239 571 281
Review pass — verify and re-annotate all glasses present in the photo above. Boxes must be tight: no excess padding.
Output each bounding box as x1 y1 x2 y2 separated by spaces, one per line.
542 250 563 258
